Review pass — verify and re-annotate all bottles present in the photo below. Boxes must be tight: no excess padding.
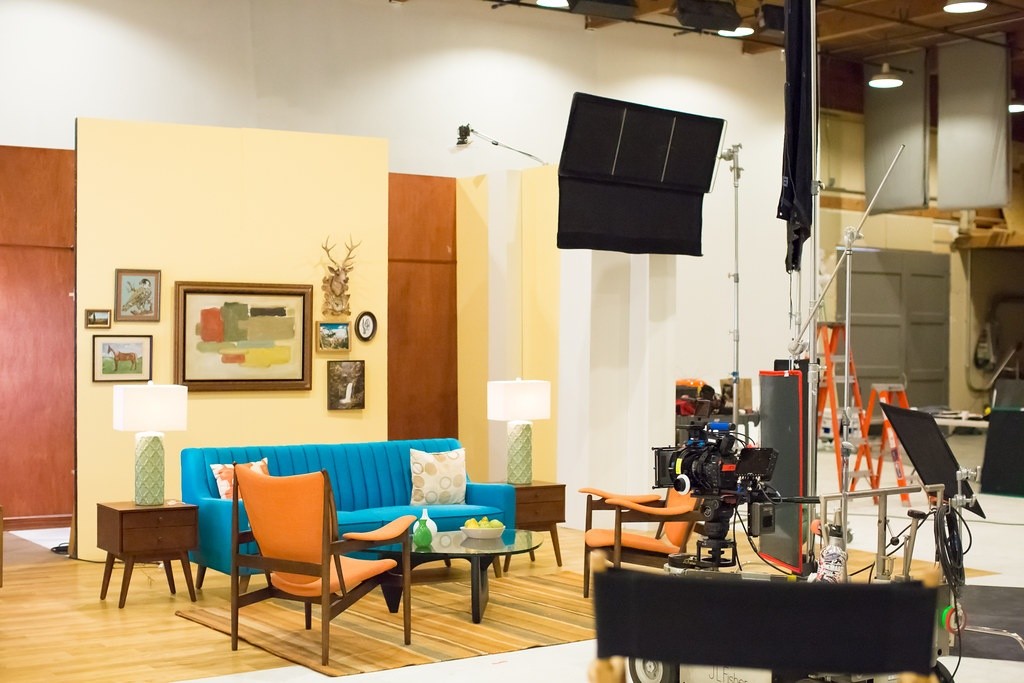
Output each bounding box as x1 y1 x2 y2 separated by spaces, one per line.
413 519 432 548
412 509 437 538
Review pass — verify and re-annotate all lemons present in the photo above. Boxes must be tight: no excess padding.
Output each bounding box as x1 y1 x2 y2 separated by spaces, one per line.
465 516 504 529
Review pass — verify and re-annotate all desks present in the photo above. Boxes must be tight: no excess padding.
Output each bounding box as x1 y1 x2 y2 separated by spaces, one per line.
362 530 544 623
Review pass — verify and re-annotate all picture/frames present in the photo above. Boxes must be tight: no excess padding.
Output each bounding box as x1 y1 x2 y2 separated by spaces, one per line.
85 309 112 329
114 268 161 322
93 335 153 382
315 321 352 351
354 311 378 341
327 360 366 409
173 280 312 392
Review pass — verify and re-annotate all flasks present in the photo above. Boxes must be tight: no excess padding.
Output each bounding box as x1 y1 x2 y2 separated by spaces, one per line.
818 524 847 584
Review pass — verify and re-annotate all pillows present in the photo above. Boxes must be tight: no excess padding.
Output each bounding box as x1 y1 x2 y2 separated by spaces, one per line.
405 447 467 504
208 457 271 500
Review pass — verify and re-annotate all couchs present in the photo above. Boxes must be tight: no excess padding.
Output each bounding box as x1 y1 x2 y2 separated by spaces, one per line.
179 439 517 594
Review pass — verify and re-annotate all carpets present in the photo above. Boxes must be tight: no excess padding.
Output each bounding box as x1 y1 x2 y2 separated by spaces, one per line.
174 560 599 678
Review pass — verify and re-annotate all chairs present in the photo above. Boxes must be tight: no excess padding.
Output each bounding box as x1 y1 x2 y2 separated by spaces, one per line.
229 460 418 667
579 489 704 598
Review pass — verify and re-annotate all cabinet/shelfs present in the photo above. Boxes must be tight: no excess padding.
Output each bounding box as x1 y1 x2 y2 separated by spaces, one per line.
97 499 200 608
502 480 566 571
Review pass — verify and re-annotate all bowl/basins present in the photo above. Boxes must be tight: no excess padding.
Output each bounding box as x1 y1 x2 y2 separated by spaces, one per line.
460 526 505 539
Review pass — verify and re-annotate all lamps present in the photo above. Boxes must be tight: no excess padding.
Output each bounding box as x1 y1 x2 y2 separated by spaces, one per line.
487 377 552 485
112 382 188 506
537 0 1024 113
